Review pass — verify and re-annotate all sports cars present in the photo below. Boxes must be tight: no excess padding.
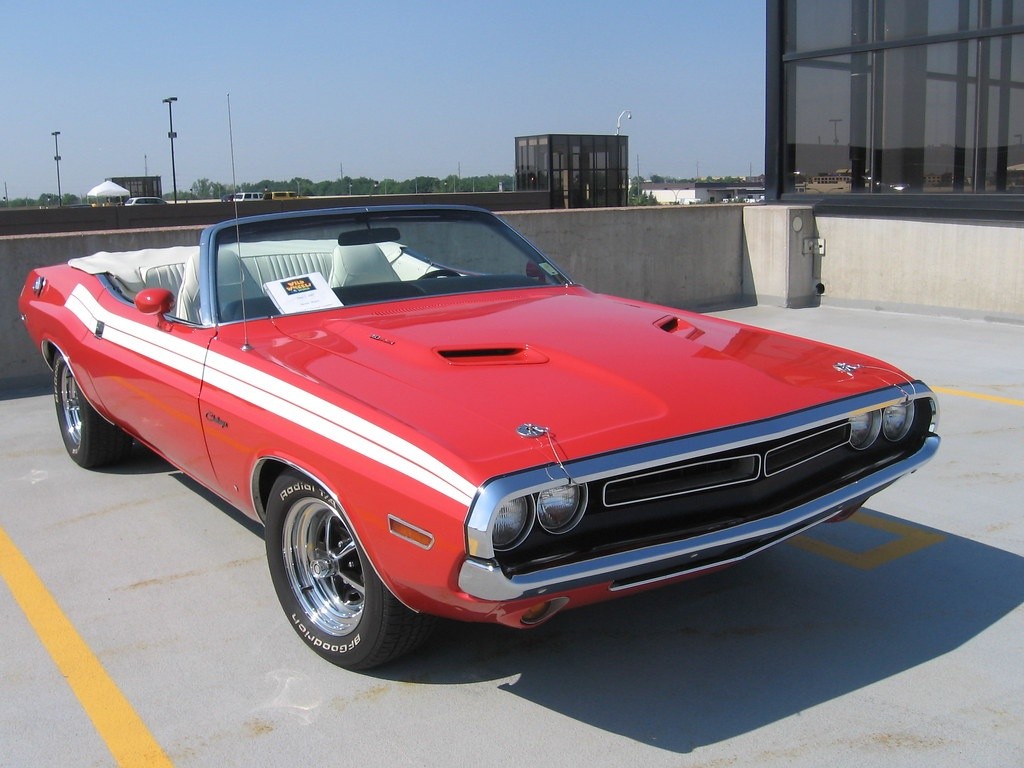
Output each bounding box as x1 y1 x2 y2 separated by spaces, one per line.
17 204 939 674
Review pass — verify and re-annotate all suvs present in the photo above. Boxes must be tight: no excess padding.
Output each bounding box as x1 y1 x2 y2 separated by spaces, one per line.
221 194 235 203
271 191 311 201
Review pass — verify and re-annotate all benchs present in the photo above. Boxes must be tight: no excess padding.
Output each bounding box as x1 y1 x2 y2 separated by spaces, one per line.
125 241 398 314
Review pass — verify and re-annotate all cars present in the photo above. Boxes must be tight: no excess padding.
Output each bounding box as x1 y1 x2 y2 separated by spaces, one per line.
124 196 168 206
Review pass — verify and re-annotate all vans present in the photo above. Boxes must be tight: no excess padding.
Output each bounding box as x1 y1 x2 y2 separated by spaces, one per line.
233 191 265 201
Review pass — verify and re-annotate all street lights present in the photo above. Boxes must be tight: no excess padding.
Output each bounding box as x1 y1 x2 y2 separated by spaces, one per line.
52 131 61 208
163 96 177 204
616 109 632 135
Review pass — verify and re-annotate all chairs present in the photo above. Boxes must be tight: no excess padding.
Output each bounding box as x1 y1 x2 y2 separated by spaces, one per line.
177 248 263 327
328 241 401 291
